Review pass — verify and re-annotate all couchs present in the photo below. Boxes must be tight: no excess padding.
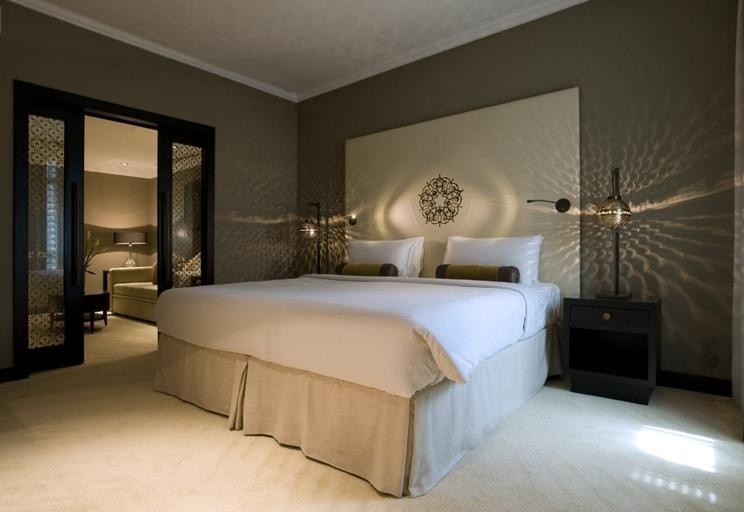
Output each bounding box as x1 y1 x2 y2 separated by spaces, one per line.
106 265 159 323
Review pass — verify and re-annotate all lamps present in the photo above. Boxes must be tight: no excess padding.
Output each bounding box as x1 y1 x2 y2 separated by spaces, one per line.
300 202 321 274
113 231 148 267
596 166 635 300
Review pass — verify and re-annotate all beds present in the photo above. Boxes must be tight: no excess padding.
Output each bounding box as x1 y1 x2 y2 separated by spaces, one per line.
152 277 560 498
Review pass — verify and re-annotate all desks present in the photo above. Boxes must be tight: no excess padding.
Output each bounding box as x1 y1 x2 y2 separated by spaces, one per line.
47 291 110 334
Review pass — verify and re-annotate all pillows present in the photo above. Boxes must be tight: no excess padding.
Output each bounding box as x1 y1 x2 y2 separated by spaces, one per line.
336 234 545 284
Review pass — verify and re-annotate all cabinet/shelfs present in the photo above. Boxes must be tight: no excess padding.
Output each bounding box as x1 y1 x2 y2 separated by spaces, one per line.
560 294 663 406
